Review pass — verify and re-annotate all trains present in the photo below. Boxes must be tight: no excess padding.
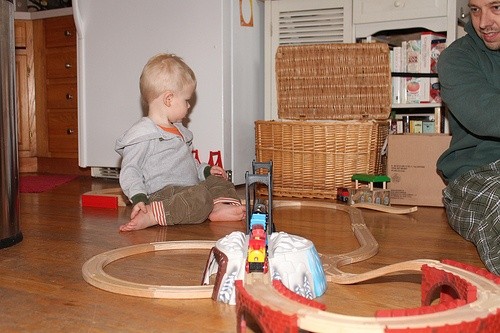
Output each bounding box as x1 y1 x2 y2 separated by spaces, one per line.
245 204 268 274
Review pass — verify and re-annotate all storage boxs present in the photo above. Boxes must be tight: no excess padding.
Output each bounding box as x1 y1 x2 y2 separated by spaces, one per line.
390 31 447 104
423 122 435 133
410 120 423 134
386 132 453 208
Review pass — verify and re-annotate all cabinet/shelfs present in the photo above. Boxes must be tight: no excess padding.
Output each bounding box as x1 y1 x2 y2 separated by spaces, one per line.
351 0 459 138
11 16 91 178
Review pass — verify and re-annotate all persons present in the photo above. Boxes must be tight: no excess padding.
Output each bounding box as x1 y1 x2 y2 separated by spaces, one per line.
114 54 246 232
436 0 500 277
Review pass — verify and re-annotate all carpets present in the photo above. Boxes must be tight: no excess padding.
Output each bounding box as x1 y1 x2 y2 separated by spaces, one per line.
19 170 78 194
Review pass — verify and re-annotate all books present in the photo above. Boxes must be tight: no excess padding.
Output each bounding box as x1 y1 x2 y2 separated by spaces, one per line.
361 32 446 104
